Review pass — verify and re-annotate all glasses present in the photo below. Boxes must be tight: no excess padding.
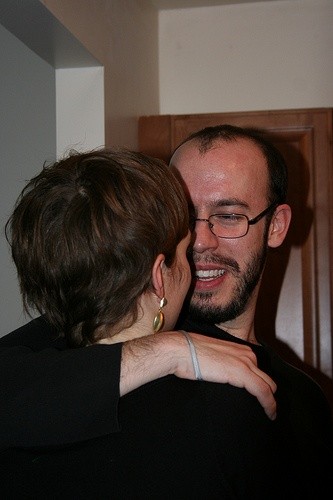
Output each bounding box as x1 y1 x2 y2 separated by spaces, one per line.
188 202 277 239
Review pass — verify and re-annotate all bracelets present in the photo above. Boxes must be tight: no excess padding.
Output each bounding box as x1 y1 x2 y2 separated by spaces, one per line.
179 330 202 380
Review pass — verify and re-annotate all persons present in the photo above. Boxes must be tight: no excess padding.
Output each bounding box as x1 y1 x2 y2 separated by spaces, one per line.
0 125 333 449
0 146 333 500
